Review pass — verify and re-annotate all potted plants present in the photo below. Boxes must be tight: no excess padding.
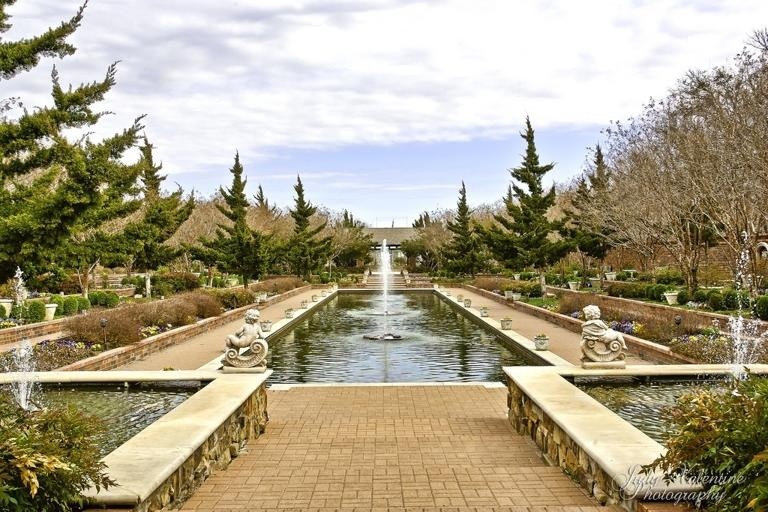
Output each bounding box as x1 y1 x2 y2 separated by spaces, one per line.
498 280 528 300
534 332 551 351
300 282 336 309
500 315 513 330
605 272 616 280
567 274 581 290
571 259 583 276
663 290 678 305
253 283 268 303
434 281 490 318
261 321 273 331
285 307 294 318
589 278 601 287
513 273 520 280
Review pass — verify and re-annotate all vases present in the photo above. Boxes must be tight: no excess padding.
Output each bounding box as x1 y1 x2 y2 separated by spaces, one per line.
41 304 58 321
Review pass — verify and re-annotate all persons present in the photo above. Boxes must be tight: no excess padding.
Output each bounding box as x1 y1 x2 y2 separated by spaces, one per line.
581 304 628 352
224 309 263 354
